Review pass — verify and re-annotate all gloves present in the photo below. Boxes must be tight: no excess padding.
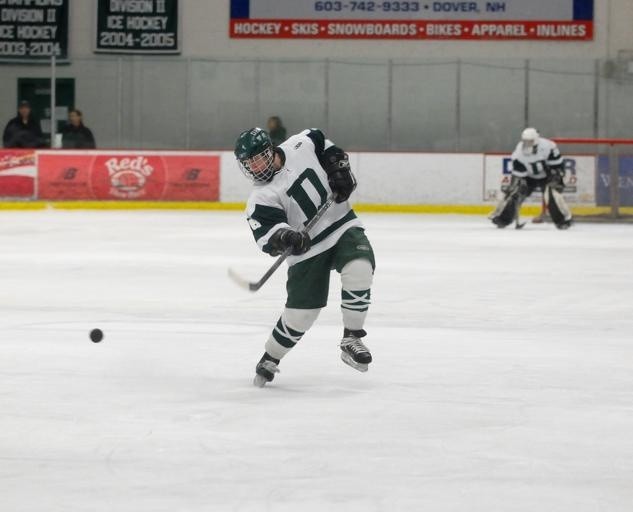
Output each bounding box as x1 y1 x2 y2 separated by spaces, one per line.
323 151 357 204
261 227 311 258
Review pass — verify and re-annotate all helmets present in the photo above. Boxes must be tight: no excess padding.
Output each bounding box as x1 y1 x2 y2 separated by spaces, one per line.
521 128 540 147
233 126 273 163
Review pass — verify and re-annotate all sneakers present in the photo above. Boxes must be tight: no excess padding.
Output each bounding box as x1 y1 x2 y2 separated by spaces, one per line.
254 351 280 383
338 327 373 364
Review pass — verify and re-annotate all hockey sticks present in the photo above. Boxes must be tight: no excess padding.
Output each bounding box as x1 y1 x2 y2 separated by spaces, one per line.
228 193 335 292
512 191 527 229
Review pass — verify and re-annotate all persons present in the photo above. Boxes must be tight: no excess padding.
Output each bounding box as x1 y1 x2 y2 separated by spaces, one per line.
60 109 97 148
487 126 574 230
233 125 376 383
3 98 43 148
268 116 286 146
